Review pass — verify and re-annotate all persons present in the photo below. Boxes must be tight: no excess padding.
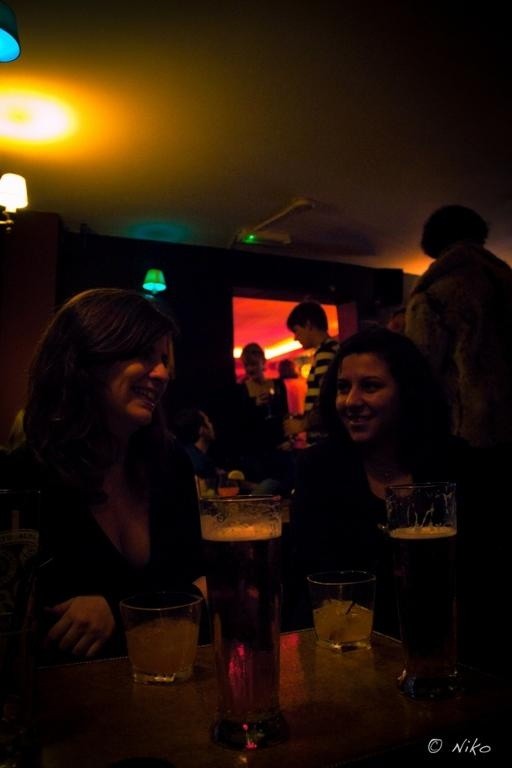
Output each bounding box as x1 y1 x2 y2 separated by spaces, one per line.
0 207 512 665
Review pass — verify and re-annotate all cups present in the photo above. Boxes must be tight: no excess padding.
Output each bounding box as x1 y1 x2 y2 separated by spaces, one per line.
304 570 380 650
115 590 209 681
131 675 204 739
194 491 291 756
380 480 465 705
313 642 373 707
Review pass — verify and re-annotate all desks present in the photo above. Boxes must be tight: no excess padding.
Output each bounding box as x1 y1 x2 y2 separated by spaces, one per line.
43 614 510 765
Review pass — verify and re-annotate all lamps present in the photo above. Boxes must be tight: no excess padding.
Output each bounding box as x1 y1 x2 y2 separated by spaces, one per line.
142 265 168 295
0 0 23 66
0 172 31 229
